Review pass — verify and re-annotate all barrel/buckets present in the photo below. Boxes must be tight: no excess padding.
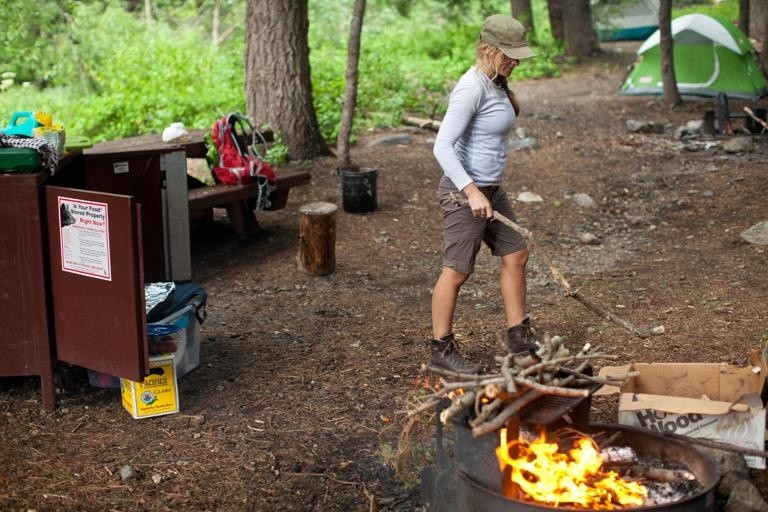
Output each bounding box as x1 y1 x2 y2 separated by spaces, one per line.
341 163 377 212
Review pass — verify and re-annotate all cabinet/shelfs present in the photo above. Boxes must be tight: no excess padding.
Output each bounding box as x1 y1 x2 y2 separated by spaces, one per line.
0 147 193 412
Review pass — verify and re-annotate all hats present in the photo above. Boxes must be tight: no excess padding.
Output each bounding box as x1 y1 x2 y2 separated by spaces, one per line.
479 14 537 60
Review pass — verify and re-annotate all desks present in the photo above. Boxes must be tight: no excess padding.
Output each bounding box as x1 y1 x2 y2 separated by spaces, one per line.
82 128 274 250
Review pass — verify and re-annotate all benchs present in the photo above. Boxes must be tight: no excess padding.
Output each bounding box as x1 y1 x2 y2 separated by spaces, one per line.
189 166 311 243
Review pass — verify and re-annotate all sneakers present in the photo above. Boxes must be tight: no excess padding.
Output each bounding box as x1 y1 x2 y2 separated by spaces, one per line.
506 314 545 353
430 332 481 374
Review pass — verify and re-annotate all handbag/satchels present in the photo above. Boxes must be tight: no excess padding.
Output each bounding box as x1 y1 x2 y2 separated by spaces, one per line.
144 280 207 323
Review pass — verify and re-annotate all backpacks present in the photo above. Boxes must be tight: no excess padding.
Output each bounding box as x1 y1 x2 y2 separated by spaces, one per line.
211 110 278 213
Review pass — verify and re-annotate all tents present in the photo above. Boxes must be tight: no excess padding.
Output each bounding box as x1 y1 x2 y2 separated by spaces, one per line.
616 13 763 99
585 0 662 43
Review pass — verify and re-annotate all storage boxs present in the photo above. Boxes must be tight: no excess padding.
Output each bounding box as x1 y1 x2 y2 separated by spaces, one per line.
589 340 768 472
86 301 202 419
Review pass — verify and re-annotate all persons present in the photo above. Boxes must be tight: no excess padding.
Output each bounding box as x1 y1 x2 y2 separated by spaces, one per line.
428 11 542 376
